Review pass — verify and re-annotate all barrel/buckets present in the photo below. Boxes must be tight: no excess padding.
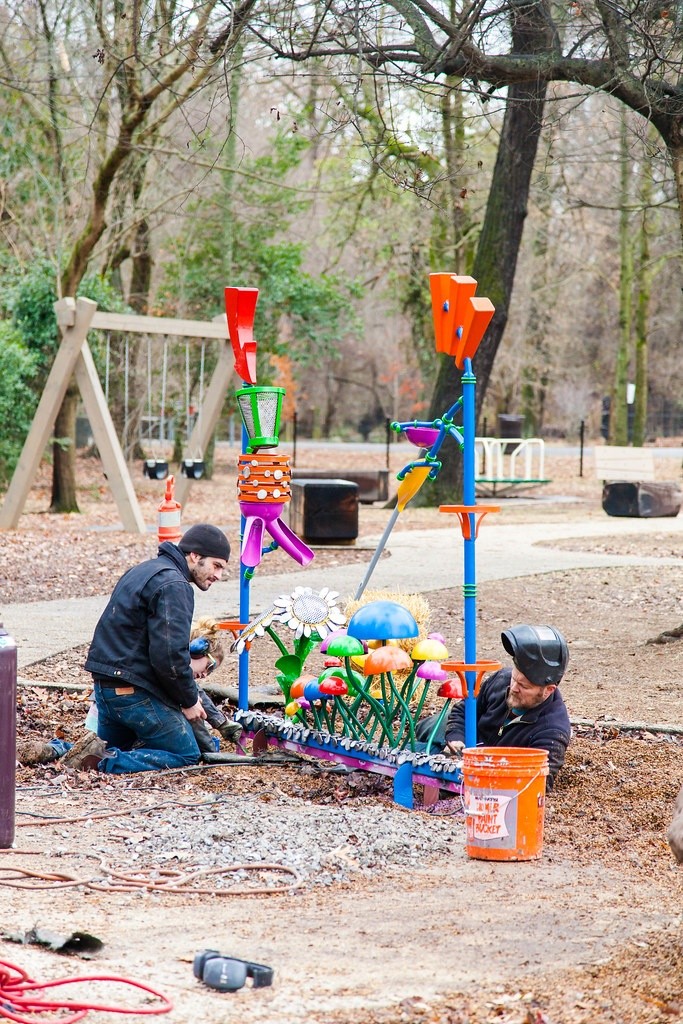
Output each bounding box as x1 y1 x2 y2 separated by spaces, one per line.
461 747 550 861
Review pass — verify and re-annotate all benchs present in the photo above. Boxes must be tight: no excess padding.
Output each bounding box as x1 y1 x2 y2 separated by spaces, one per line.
287 477 358 547
595 444 654 485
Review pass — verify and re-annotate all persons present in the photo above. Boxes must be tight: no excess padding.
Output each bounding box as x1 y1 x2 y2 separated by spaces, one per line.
406 626 571 795
16 524 231 775
85 618 242 753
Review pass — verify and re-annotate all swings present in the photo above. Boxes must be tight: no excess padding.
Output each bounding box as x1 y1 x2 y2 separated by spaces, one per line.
144 333 169 479
103 332 130 481
181 336 206 480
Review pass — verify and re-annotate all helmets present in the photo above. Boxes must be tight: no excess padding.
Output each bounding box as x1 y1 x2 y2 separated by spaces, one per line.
501 625 568 686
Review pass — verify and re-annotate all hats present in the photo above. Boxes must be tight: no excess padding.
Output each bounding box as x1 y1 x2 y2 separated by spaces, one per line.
178 524 231 562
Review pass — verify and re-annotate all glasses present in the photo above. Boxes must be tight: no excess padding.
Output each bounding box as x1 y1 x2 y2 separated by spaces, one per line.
206 653 217 677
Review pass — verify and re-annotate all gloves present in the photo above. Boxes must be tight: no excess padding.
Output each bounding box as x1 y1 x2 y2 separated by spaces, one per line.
217 720 243 744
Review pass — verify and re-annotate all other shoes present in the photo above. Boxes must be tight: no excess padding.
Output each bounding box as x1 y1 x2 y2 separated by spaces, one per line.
17 741 53 765
58 732 114 769
